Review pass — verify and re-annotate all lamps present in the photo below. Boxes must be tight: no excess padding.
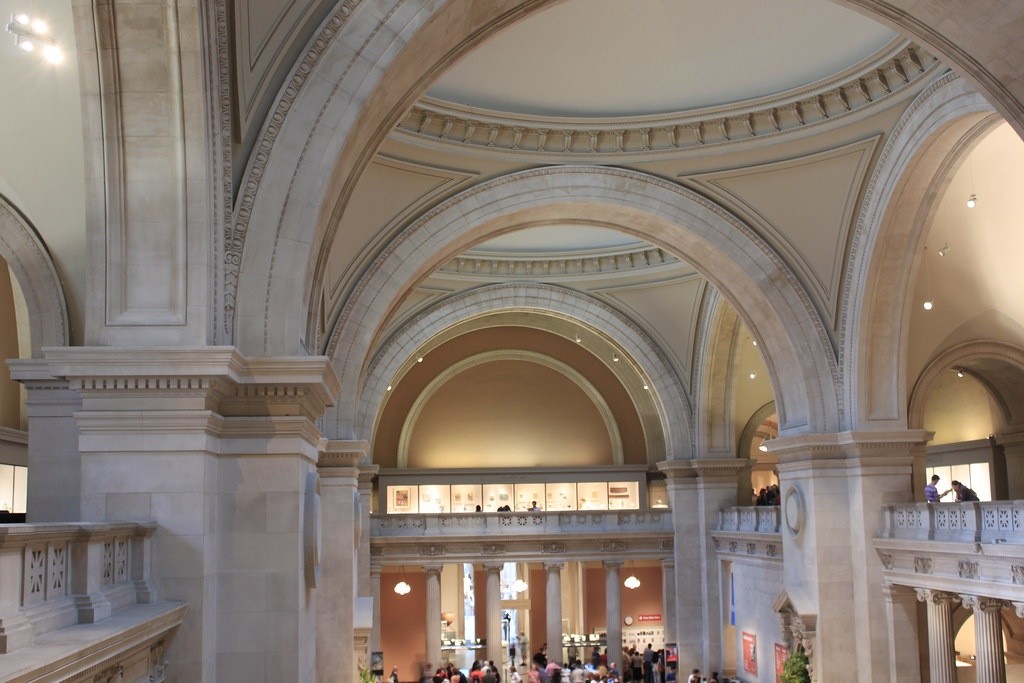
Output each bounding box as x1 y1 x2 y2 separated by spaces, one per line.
957 367 965 378
508 561 528 592
758 416 778 453
624 560 641 590
966 155 978 208
923 246 935 310
6 13 68 67
575 332 582 343
938 241 953 257
393 564 411 595
613 352 619 362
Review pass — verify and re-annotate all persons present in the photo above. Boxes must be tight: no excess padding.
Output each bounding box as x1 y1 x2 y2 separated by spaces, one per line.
387 638 733 683
952 481 979 503
475 505 481 512
529 501 540 511
503 505 511 511
924 475 949 501
498 506 504 511
751 484 780 505
502 613 511 640
516 632 527 665
